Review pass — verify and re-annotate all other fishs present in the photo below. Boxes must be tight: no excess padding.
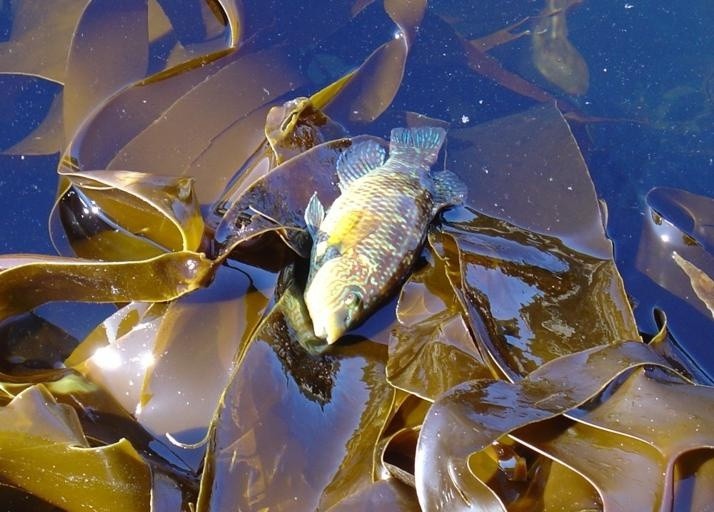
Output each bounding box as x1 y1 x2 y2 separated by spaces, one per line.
300 124 469 346
